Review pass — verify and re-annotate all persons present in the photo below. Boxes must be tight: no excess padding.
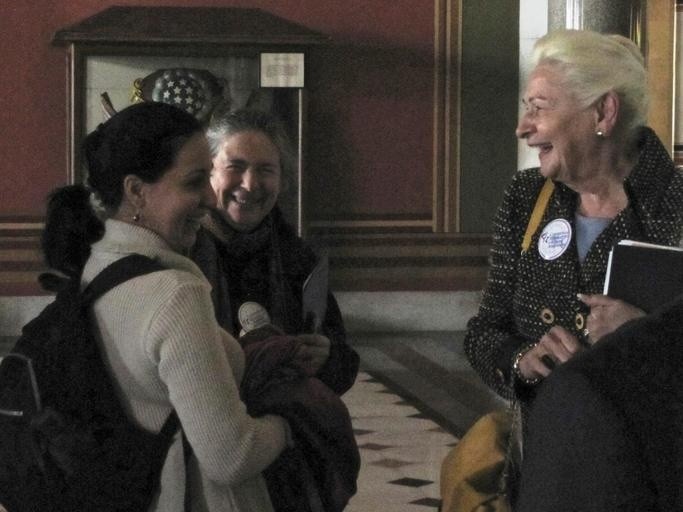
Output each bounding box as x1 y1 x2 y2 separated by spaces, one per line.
461 28 682 511
505 292 683 510
178 100 362 510
44 101 293 510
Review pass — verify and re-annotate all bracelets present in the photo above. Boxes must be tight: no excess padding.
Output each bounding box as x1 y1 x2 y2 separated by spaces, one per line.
513 341 542 386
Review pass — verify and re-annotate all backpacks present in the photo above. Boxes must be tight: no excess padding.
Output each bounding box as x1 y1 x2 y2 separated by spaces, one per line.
0 253 180 512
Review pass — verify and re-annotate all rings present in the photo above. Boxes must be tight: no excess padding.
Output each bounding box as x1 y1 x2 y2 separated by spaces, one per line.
584 329 592 337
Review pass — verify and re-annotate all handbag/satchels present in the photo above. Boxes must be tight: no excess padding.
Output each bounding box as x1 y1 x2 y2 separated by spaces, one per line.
439 409 523 512
